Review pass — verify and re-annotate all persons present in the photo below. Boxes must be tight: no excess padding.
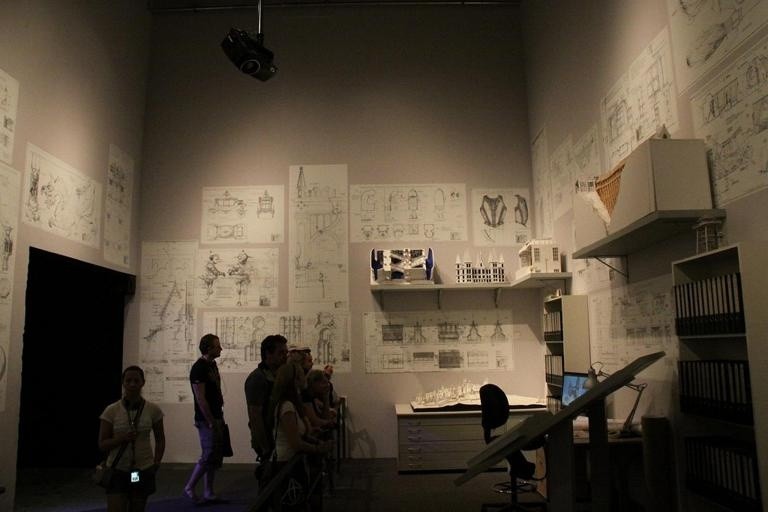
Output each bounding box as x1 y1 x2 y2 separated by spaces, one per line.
96 365 166 511
182 333 225 503
244 334 347 512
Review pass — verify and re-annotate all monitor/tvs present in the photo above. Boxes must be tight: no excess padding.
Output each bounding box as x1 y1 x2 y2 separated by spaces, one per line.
560 371 590 408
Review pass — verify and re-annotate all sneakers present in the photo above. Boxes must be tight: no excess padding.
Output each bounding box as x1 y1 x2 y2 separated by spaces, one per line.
183 486 230 505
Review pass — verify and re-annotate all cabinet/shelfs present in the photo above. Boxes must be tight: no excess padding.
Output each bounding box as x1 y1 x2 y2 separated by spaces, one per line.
541 294 591 416
393 404 548 473
671 241 767 511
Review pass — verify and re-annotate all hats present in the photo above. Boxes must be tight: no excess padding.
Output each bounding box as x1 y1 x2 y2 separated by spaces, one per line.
288 344 312 353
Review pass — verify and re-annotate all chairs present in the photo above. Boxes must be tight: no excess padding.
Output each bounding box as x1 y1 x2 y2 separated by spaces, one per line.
480 384 548 511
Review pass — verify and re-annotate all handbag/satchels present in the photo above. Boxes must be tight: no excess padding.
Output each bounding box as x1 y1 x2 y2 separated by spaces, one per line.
222 423 234 458
90 464 113 486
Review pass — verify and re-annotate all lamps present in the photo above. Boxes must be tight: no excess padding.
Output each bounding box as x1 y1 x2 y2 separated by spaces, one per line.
590 362 648 439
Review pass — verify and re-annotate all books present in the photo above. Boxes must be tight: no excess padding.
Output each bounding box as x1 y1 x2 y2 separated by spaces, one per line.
543 311 564 416
674 271 759 500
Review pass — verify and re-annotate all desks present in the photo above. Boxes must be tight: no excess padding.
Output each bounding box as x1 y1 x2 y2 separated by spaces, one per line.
536 424 643 512
